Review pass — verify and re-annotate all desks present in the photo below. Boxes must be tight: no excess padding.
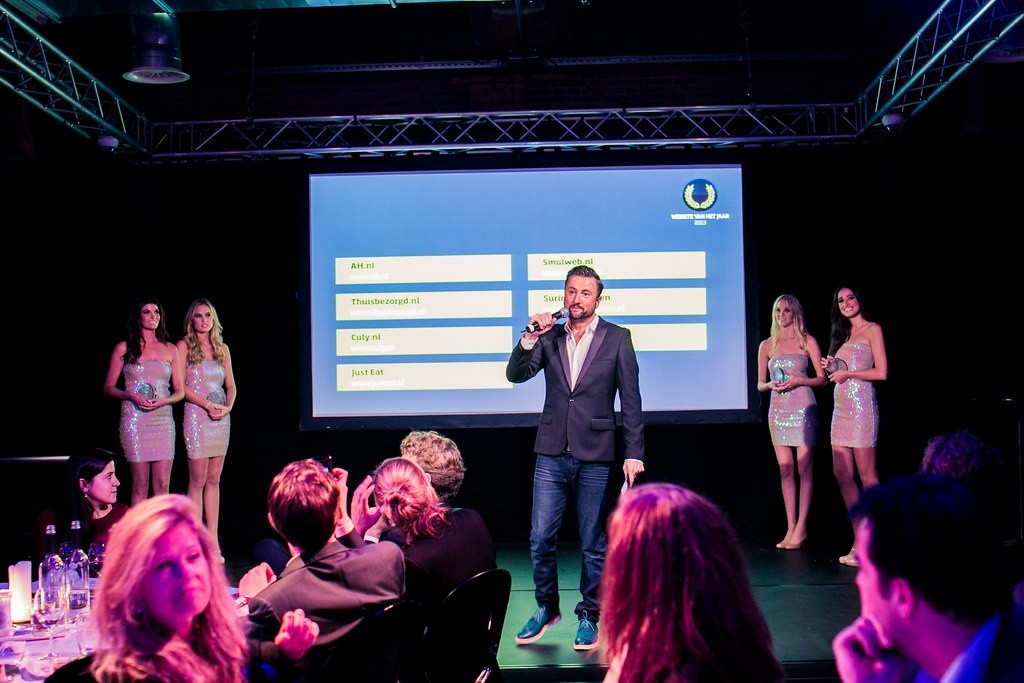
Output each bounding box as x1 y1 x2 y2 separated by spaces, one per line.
0 578 240 683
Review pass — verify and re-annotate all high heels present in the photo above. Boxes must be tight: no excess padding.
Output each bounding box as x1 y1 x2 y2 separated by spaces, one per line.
776 531 806 549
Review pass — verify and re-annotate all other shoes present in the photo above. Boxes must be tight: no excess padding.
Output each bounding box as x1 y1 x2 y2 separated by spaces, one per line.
839 546 861 565
219 549 224 563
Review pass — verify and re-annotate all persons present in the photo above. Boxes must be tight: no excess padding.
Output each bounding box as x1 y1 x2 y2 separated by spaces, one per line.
104 302 185 508
757 294 827 549
506 265 644 649
821 284 887 567
42 431 1024 683
176 300 236 564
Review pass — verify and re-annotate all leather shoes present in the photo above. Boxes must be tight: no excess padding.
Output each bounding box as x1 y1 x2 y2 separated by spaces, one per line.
514 605 562 644
575 612 599 650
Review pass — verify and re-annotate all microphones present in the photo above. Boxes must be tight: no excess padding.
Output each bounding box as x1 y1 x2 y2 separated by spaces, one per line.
521 307 569 333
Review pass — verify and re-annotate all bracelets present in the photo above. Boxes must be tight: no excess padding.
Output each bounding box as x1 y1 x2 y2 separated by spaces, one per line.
205 401 211 408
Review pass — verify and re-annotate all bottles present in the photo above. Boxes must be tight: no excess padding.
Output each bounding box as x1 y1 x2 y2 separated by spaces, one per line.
37 524 66 616
88 543 105 577
63 520 91 614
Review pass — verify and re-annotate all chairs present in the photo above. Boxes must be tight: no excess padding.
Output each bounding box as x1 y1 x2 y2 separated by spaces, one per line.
279 568 511 683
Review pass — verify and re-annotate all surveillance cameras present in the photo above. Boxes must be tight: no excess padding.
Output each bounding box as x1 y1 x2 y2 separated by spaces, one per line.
882 114 903 131
98 135 119 152
